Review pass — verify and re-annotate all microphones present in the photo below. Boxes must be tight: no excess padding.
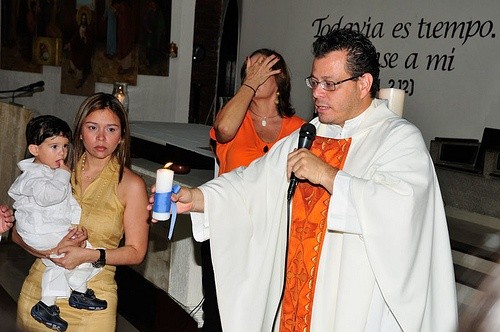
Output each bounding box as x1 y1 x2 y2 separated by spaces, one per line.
287 122 316 202
14 80 45 94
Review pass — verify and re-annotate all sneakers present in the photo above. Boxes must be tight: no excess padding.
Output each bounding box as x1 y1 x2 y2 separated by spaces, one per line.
69 289 107 310
30 301 68 332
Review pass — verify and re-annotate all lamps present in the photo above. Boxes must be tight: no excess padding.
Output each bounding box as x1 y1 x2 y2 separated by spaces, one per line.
113 81 129 114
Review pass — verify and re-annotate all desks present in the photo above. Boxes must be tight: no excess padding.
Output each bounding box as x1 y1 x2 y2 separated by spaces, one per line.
124 124 499 332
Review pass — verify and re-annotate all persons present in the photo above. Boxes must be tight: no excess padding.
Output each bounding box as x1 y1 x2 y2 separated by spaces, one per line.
0 205 15 241
210 48 307 177
148 29 458 332
8 115 107 332
11 93 151 332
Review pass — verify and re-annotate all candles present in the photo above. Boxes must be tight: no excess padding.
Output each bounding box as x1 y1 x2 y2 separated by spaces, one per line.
152 162 174 222
380 88 405 118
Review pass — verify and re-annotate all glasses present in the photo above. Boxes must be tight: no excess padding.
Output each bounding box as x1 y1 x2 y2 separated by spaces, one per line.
304 75 359 91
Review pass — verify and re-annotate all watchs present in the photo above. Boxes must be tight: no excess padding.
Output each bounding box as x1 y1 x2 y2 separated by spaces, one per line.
92 249 106 268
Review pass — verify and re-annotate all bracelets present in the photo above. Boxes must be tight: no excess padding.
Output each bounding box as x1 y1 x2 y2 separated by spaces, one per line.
243 84 256 92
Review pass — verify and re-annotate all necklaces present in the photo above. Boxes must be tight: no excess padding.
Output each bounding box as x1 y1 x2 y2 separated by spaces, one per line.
82 156 95 181
250 108 279 126
